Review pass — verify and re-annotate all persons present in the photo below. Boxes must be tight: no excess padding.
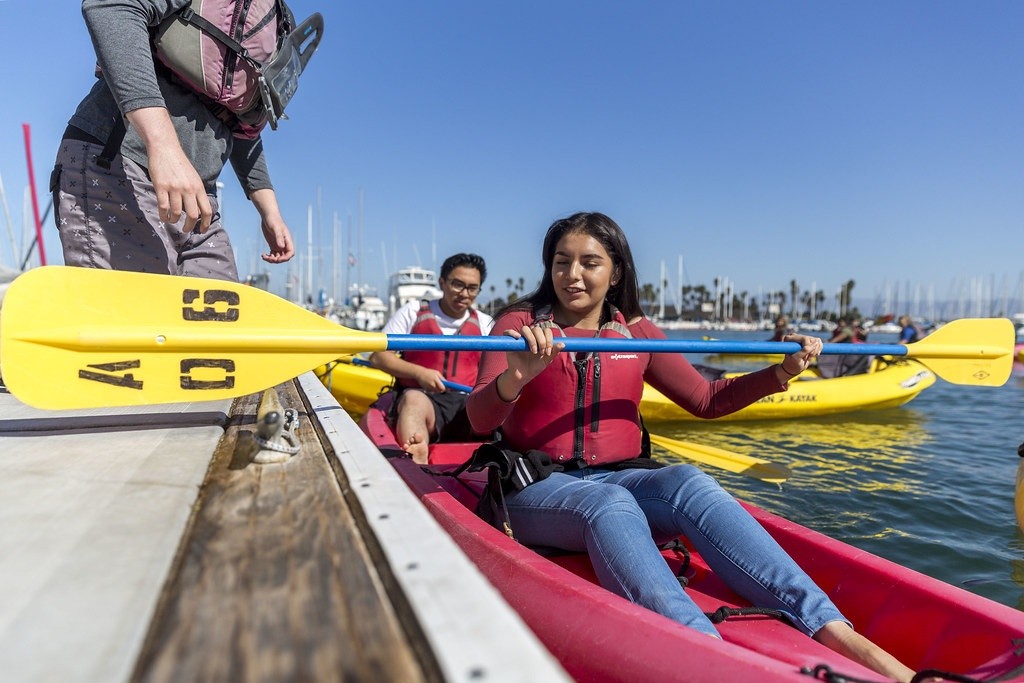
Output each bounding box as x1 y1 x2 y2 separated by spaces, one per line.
898 315 919 344
468 212 942 683
369 254 497 467
50 0 324 282
772 316 787 342
825 307 867 343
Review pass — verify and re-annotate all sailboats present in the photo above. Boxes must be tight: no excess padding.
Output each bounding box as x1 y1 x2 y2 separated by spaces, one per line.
648 254 1024 349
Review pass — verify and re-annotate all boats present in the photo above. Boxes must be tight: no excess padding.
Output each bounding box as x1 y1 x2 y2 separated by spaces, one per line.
299 283 389 334
366 384 1024 683
387 266 440 321
638 347 938 424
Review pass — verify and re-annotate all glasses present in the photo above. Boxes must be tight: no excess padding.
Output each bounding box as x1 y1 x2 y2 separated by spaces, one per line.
446 277 480 296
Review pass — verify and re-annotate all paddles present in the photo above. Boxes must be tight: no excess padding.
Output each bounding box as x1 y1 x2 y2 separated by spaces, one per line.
870 311 895 327
0 259 1021 415
336 354 798 487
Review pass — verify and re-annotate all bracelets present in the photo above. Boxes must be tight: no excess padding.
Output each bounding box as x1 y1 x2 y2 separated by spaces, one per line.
782 364 798 376
